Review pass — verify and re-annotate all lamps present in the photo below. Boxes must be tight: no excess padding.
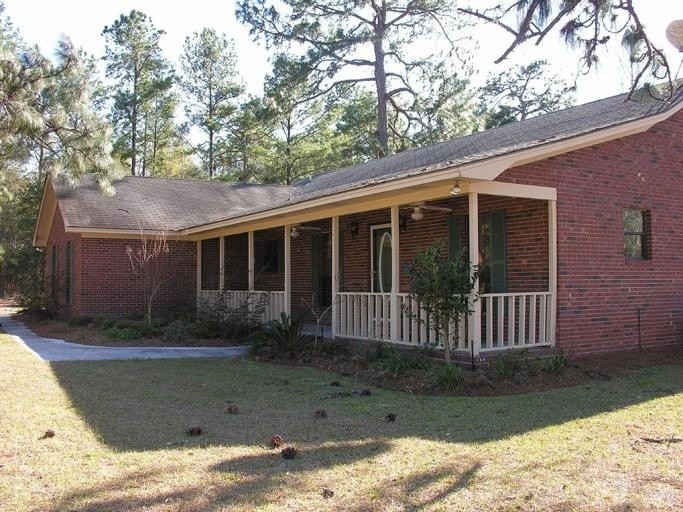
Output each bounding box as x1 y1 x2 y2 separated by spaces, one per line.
410 207 424 222
290 228 300 238
449 179 463 198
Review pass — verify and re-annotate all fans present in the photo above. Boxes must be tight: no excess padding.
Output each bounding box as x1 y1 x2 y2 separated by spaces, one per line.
399 202 453 222
289 224 324 238
